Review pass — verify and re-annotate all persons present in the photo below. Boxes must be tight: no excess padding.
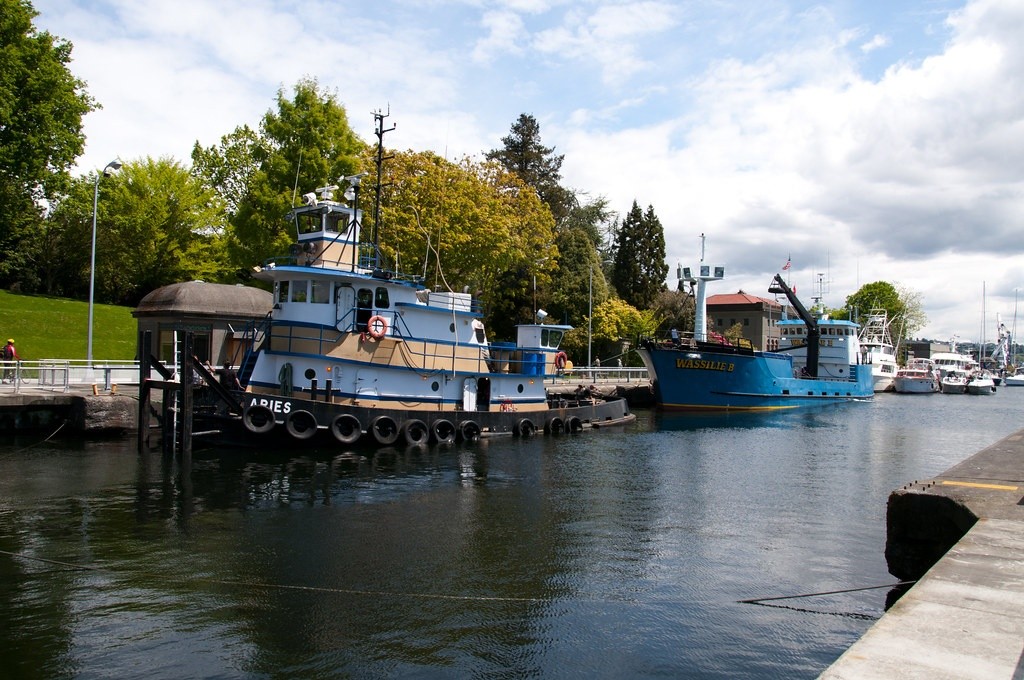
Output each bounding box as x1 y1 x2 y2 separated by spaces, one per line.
927 363 932 375
2 339 17 379
670 324 679 347
206 360 245 391
860 344 868 366
594 356 602 378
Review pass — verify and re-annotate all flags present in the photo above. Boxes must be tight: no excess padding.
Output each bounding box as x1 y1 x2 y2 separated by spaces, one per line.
781 258 791 270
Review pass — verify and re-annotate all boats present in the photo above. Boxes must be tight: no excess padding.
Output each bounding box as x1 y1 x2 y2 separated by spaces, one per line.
213 101 637 450
634 232 875 415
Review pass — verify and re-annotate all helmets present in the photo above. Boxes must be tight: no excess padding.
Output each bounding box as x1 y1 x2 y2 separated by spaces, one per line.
8 338 14 343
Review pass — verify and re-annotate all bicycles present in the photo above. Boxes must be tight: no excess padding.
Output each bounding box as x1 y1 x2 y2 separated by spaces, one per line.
2 359 33 384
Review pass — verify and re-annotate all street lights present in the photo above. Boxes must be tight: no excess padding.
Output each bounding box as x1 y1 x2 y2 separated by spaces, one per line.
83 157 122 386
587 261 617 379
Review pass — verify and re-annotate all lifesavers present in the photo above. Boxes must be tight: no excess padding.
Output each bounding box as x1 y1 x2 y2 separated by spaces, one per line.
243 405 276 434
567 416 583 432
432 418 457 445
286 409 317 439
330 414 362 443
367 416 400 446
368 315 387 338
555 351 568 368
401 418 429 445
459 419 481 441
548 416 566 435
513 417 536 438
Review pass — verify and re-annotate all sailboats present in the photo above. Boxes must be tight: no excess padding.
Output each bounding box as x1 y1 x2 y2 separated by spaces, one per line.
857 281 1024 395
